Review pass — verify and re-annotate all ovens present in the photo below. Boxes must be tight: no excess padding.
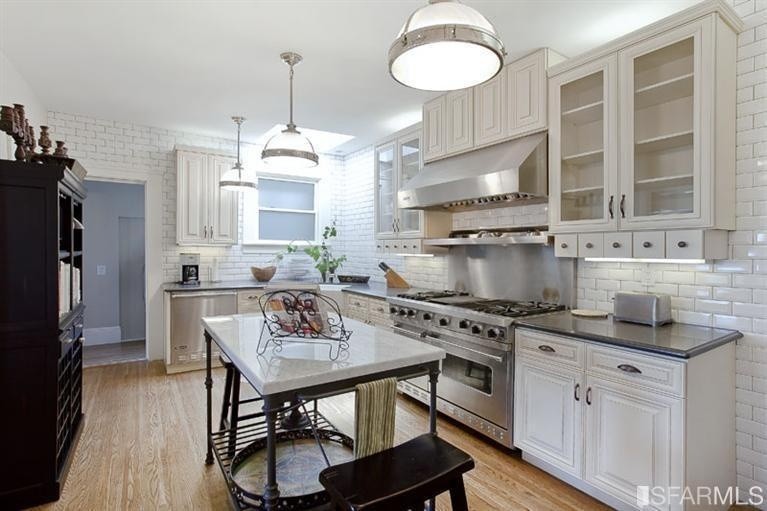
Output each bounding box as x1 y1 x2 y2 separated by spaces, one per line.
391 297 513 430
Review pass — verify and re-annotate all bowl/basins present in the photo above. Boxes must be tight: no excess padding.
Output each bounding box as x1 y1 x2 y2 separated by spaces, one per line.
338 275 370 283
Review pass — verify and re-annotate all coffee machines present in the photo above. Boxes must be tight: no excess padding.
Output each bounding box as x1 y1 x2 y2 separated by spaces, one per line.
179 252 199 284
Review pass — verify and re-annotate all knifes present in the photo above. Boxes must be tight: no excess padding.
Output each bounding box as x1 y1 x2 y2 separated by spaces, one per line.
378 262 390 272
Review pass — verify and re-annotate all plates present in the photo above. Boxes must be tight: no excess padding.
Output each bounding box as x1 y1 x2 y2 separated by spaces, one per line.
570 309 608 317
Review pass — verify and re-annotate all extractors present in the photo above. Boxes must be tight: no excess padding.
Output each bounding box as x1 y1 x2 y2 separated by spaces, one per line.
397 130 548 212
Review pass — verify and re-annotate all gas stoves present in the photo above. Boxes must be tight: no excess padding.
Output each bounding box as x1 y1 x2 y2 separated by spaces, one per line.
386 289 567 326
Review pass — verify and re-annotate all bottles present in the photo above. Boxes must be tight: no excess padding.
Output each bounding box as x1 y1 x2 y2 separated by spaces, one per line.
212 258 219 282
572 191 594 219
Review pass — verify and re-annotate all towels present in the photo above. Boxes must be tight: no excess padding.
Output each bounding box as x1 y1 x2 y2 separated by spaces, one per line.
353 376 399 461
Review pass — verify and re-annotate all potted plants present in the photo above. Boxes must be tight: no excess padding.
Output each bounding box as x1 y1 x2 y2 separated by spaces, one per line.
267 216 347 283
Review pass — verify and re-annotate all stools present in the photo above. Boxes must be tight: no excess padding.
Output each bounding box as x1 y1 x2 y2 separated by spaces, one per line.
218 351 286 458
316 432 476 511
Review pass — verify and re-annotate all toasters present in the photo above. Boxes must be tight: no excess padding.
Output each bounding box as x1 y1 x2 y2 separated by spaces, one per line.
614 291 671 327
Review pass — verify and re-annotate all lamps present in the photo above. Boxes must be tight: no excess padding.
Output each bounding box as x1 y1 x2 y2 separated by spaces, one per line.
385 1 510 94
217 116 259 194
258 52 320 170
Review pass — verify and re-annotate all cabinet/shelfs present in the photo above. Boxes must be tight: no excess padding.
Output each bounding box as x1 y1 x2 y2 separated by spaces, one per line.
343 293 390 332
237 290 265 314
0 158 90 510
420 47 570 167
546 0 743 265
172 143 239 251
513 327 739 511
318 291 342 315
370 121 451 257
